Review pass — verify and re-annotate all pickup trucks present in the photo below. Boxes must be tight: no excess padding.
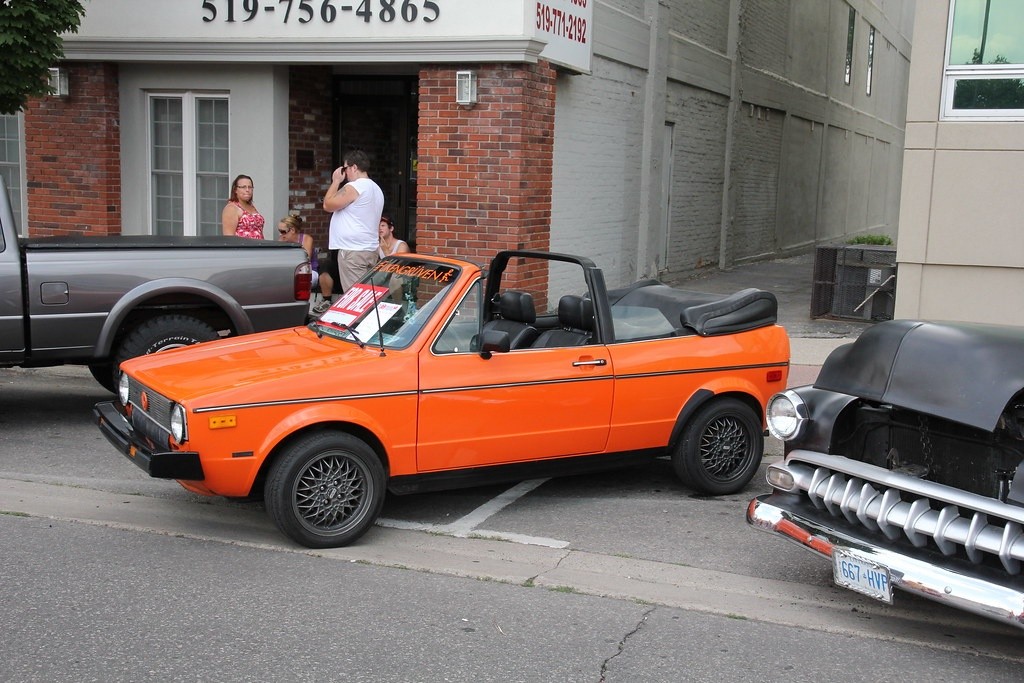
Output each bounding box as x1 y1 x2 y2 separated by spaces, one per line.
0 176 311 394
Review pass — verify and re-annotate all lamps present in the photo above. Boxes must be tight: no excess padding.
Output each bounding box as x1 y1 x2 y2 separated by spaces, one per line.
46 67 69 102
456 71 477 109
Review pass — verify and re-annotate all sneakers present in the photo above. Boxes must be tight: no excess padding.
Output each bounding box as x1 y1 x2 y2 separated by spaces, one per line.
313 300 333 313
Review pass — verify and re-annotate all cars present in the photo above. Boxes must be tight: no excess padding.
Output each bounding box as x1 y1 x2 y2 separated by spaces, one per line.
92 250 790 549
747 317 1024 630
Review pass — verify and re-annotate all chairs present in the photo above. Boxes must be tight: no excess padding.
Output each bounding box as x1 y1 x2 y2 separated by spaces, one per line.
528 295 592 348
483 292 537 350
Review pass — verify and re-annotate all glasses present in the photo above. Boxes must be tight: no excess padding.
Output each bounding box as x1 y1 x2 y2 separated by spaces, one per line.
342 165 350 172
236 186 254 190
278 228 290 234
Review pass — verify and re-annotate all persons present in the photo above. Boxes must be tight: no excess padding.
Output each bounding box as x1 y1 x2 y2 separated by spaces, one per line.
378 216 410 305
322 151 385 294
222 174 265 239
277 214 335 313
